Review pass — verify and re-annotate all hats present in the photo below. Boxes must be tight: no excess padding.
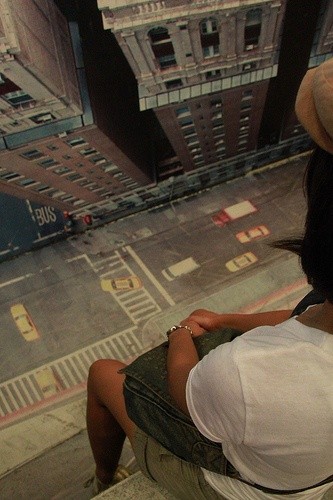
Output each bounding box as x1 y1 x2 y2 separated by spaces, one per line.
295 56 333 154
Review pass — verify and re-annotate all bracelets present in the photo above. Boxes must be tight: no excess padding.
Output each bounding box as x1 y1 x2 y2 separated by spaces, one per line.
167 321 193 338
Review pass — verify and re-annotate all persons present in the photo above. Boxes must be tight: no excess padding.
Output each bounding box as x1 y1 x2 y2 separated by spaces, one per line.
84 59 333 500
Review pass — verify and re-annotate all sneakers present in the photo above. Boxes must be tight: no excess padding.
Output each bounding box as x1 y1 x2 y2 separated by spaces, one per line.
82 465 129 495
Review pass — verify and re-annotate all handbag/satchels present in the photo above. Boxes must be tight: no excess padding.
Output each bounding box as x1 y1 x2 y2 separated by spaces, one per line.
116 325 243 479
